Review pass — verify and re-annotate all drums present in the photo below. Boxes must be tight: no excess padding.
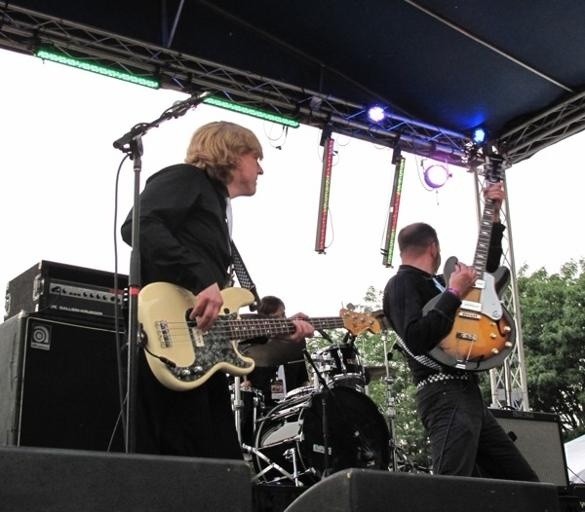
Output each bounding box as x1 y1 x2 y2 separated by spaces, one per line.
227 388 268 462
253 386 393 487
313 343 367 392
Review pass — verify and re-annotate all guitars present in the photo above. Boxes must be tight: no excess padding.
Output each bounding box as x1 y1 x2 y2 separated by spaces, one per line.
420 141 516 372
137 282 381 391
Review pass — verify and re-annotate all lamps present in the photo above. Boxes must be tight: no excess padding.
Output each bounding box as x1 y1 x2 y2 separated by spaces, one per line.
472 129 488 144
367 104 386 123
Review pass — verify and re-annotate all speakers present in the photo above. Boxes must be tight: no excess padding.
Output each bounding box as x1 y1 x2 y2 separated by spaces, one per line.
281 467 561 512
0 310 237 459
0 447 252 512
487 409 571 494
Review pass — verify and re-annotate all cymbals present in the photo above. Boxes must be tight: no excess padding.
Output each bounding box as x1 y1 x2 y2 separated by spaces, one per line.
366 368 400 381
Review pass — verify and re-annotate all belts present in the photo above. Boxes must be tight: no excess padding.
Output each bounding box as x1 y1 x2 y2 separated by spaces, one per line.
416 373 470 389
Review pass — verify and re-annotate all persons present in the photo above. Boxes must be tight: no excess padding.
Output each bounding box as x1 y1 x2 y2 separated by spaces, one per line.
120 117 317 463
381 179 541 483
229 295 308 390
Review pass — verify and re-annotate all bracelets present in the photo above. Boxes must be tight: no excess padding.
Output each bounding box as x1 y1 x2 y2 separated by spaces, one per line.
445 287 461 297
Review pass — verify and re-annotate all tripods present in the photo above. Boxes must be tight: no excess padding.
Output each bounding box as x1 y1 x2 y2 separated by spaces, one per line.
233 376 305 488
381 332 417 474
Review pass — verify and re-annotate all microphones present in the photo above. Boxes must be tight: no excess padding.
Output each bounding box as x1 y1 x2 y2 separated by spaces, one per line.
172 90 212 116
